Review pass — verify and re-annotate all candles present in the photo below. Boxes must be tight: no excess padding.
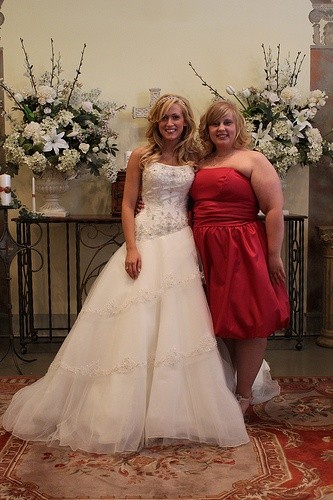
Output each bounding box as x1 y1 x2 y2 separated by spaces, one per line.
124 151 132 168
0 173 11 206
32 176 36 214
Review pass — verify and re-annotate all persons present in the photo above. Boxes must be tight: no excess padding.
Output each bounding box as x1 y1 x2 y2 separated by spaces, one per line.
0 95 250 454
137 101 290 423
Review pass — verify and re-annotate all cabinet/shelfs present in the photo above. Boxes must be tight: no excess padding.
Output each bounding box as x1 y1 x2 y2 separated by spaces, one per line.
110 171 142 218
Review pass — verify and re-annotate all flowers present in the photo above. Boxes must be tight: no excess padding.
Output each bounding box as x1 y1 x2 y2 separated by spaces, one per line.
0 35 126 183
189 44 333 175
36 173 71 217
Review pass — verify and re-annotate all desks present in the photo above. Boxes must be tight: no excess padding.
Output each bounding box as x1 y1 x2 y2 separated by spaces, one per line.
266 213 309 350
10 214 125 357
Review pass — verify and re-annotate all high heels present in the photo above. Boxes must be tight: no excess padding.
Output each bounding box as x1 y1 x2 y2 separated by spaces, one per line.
233 391 254 416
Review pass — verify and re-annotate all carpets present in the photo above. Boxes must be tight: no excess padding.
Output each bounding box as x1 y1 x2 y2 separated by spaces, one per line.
0 375 333 500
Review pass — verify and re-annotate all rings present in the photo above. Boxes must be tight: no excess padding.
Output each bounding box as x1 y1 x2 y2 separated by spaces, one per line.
125 267 128 270
